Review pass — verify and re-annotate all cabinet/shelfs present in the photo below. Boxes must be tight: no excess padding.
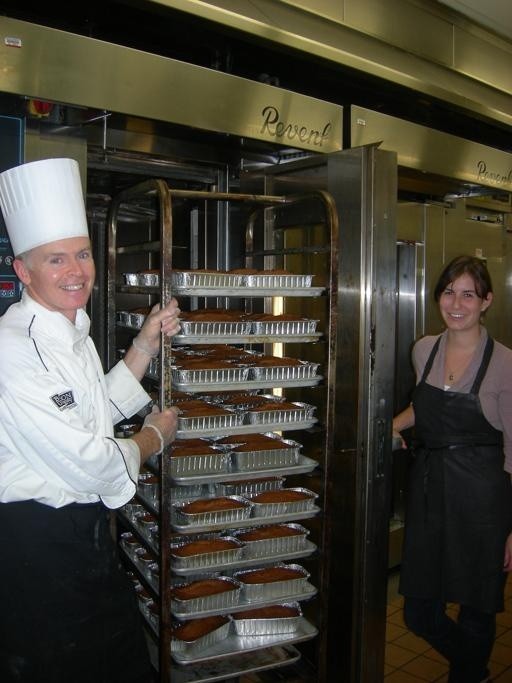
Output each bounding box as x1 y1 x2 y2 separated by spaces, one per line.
106 178 342 683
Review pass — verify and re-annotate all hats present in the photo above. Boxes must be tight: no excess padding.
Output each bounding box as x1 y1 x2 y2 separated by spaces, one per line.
1 156 89 257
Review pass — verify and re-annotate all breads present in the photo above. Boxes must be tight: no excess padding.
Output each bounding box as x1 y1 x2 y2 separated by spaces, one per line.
126 266 315 642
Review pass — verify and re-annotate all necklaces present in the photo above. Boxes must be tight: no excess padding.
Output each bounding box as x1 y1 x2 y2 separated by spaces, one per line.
445 353 472 380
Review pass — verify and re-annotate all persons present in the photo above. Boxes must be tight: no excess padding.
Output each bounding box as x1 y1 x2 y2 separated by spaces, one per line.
0 235 182 682
393 256 512 682
